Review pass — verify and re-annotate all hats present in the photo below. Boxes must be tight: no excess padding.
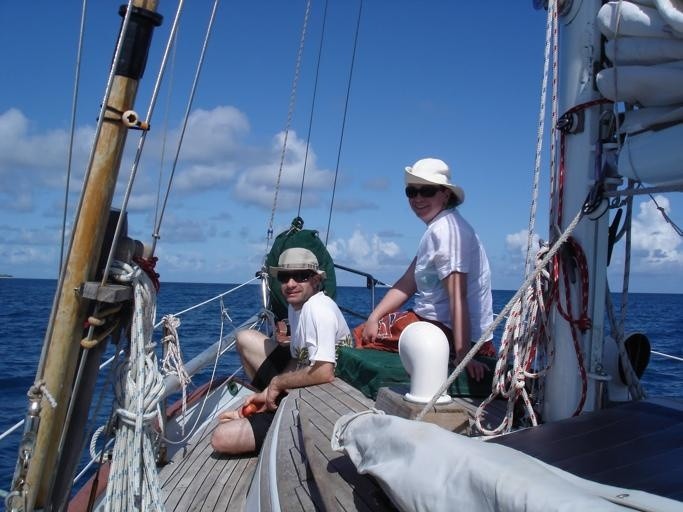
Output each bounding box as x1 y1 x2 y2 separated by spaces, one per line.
403 157 466 205
268 246 328 281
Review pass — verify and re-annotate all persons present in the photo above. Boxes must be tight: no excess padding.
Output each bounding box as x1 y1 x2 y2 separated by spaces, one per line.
207 246 354 455
359 156 497 381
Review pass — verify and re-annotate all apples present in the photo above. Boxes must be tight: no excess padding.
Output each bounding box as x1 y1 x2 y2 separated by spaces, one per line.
243 404 256 417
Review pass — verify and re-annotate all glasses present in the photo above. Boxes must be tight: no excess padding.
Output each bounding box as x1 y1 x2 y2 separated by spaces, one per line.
276 269 316 283
404 185 449 199
